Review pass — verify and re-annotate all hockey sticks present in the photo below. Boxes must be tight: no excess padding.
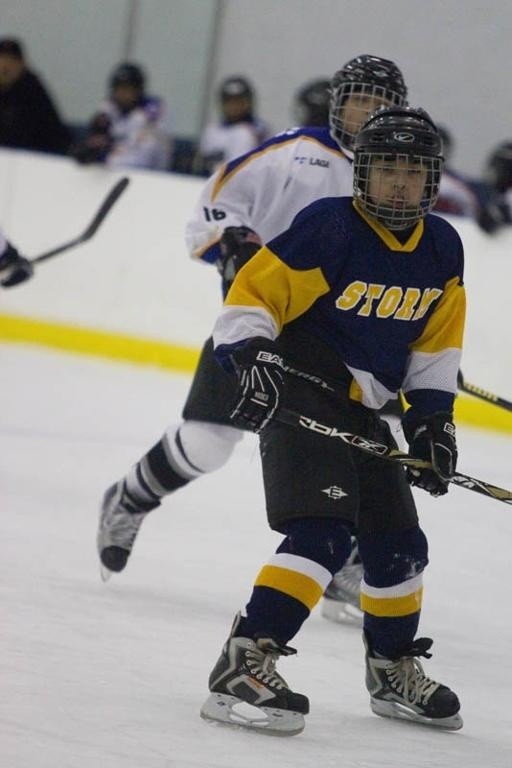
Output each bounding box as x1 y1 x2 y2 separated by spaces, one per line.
1 178 128 282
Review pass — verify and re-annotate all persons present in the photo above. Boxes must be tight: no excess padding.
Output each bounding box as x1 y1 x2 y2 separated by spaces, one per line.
190 77 268 178
86 64 172 173
95 55 409 630
298 79 333 128
0 37 512 234
199 104 464 734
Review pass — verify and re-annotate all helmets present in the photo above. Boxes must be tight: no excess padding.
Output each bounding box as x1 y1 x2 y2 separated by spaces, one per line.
300 54 443 208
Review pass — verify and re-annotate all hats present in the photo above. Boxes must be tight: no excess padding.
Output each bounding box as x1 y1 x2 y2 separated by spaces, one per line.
112 65 247 97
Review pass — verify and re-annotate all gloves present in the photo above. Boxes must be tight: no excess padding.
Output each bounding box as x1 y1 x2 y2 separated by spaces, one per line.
224 336 289 434
221 225 263 286
403 413 458 497
0 243 33 287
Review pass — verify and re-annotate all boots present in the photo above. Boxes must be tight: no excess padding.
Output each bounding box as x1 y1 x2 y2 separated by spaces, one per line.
96 477 161 573
323 569 363 610
208 611 310 715
362 629 461 719
